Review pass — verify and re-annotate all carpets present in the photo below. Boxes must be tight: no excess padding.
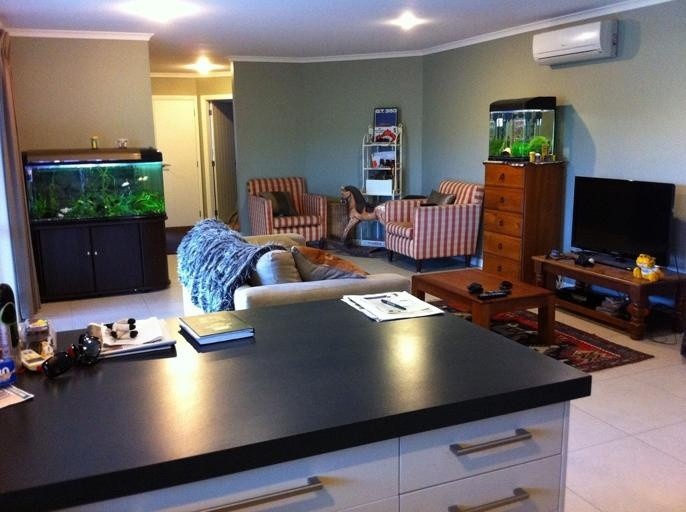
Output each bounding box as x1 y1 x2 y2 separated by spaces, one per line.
426 300 654 372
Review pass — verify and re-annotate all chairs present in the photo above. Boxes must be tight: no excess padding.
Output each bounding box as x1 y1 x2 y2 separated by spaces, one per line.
248 177 328 251
386 182 483 272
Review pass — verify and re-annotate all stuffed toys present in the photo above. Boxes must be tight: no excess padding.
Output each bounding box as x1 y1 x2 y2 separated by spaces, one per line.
633 254 665 282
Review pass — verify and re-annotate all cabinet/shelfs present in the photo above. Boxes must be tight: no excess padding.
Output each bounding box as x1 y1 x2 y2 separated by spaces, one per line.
481 160 566 283
359 124 402 249
30 215 169 303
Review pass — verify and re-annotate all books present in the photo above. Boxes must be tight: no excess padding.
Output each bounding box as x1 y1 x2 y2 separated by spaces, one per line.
97 316 177 359
179 311 255 345
595 295 630 316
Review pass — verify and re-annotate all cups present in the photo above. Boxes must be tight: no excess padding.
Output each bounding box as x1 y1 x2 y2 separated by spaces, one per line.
113 139 128 150
547 144 553 155
21 318 49 357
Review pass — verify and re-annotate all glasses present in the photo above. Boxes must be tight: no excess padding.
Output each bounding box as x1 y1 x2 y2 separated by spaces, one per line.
42 344 78 380
79 335 101 365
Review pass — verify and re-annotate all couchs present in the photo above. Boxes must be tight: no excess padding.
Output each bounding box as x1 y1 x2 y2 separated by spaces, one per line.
178 218 412 316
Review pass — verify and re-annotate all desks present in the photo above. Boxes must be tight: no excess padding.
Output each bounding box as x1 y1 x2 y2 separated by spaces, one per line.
531 255 686 341
2 291 592 512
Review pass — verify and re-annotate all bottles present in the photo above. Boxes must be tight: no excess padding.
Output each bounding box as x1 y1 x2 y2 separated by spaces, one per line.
529 151 535 162
535 153 541 163
91 136 99 150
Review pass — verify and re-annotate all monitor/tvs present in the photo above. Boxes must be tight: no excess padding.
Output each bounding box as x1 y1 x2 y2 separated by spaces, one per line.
570 175 676 267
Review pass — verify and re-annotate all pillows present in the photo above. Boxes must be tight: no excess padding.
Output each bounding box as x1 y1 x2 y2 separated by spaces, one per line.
427 187 457 204
292 245 380 286
261 191 299 212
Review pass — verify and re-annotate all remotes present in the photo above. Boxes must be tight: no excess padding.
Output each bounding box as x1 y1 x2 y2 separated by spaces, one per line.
477 288 509 299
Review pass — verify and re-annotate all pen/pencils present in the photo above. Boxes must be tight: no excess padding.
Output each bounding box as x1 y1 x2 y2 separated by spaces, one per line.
381 299 406 310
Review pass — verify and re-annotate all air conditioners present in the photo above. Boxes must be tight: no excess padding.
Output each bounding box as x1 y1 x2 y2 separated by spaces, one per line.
532 21 617 66
